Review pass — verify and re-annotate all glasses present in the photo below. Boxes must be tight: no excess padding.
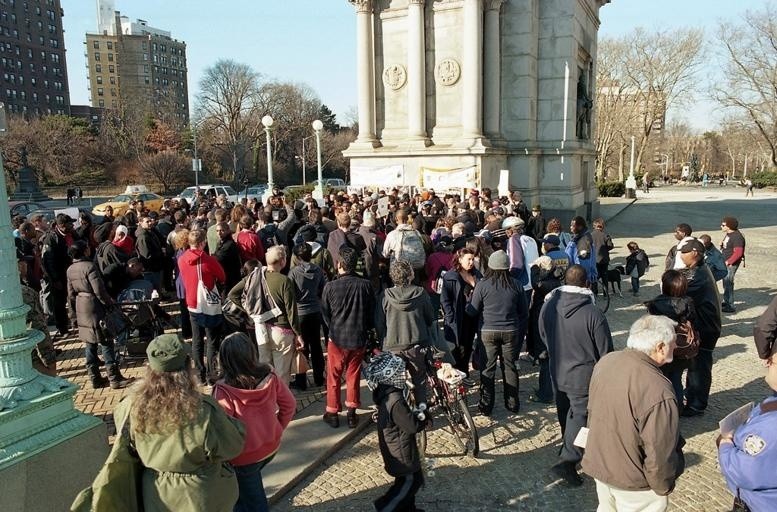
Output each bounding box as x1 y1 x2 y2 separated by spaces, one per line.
765 357 777 366
722 225 725 227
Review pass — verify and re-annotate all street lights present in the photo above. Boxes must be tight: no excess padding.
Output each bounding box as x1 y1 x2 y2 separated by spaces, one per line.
312 120 324 188
261 115 274 195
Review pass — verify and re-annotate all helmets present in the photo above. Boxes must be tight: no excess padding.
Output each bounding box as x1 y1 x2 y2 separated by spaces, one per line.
502 216 525 230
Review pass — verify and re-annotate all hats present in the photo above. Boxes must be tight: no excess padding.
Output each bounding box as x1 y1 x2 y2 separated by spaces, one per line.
528 256 554 271
146 333 192 372
487 250 511 270
539 235 560 246
677 240 706 253
57 215 77 225
367 351 411 400
114 224 128 242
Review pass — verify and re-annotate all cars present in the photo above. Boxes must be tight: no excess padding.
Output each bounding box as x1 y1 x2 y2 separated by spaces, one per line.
238 188 265 203
24 208 104 225
9 202 46 217
92 186 164 216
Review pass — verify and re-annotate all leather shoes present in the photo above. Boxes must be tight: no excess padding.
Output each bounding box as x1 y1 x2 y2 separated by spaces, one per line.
551 463 584 486
682 407 704 417
323 412 339 428
289 379 306 390
348 413 359 427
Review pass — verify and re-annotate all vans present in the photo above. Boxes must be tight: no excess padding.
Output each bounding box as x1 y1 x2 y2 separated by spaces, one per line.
326 179 345 191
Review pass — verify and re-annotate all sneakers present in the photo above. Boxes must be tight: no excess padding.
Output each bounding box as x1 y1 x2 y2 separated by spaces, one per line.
207 381 215 388
462 378 476 385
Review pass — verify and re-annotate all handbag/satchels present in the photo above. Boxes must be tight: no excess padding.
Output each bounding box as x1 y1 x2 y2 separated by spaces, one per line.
290 349 309 375
197 257 222 315
99 309 133 342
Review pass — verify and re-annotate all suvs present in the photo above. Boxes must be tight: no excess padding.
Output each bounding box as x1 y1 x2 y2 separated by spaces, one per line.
173 185 238 207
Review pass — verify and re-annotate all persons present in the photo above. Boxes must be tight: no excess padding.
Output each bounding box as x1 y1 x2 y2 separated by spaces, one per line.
538 264 614 485
537 235 569 269
382 260 434 425
41 216 76 336
441 248 483 388
678 240 721 417
243 259 262 278
565 216 598 287
721 216 745 312
177 229 224 386
659 223 703 293
78 199 142 244
228 245 305 415
135 215 169 299
288 243 328 391
121 333 246 511
582 315 685 512
288 180 542 259
592 217 614 296
626 242 649 296
157 186 286 249
464 250 527 416
644 269 699 419
700 235 728 282
65 183 84 202
15 247 56 378
321 248 374 427
213 332 296 512
528 256 562 404
13 211 44 246
754 294 777 396
366 351 431 512
67 240 135 389
717 352 777 512
541 219 571 253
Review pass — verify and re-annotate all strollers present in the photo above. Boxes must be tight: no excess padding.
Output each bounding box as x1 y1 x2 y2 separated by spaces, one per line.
113 279 162 359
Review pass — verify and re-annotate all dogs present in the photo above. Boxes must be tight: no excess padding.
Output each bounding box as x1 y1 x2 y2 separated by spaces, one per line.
599 266 625 297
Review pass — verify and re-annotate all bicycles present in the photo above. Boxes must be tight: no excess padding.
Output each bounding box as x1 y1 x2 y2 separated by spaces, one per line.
592 280 610 313
426 344 479 457
